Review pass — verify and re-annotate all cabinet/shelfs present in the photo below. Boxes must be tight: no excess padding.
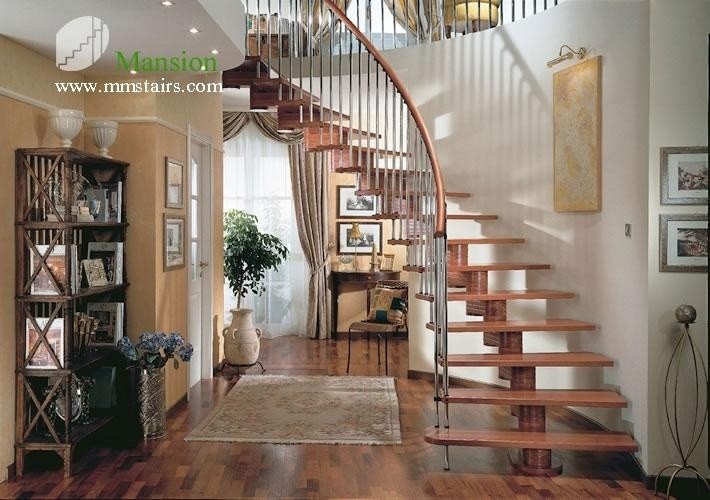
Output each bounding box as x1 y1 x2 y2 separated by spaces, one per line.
249 33 301 58
15 146 131 479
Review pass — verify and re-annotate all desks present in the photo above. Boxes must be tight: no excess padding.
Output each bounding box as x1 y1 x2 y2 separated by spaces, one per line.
330 268 402 341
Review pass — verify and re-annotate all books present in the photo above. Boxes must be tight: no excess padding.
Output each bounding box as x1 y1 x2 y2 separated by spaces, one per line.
25 180 124 370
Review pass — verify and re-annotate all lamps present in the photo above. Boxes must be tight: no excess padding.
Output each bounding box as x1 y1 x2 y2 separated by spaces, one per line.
349 222 364 271
546 41 588 69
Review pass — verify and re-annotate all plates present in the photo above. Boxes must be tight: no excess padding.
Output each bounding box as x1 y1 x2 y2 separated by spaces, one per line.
48 374 81 423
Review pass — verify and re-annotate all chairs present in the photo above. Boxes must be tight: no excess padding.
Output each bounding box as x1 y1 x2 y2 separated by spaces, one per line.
346 280 409 375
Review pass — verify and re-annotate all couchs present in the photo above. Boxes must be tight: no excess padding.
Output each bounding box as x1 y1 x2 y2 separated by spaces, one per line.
441 0 500 39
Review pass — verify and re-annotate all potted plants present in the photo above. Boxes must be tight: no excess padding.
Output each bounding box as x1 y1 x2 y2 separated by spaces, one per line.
223 208 290 365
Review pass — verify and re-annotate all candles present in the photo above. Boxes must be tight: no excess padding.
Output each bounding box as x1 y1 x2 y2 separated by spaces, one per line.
371 241 377 264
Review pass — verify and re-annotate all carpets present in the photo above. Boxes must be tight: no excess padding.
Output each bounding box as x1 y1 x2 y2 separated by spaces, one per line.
181 373 403 447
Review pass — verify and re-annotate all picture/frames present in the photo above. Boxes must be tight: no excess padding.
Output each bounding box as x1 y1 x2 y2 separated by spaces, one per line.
164 156 187 210
380 254 396 272
162 211 187 273
82 258 108 288
551 54 604 214
657 212 709 274
334 221 384 255
336 184 385 220
658 144 708 207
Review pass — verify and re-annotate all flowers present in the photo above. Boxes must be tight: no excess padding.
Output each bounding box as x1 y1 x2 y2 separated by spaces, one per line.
116 330 195 370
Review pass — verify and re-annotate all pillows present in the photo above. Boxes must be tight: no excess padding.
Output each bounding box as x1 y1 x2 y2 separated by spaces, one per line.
365 287 407 325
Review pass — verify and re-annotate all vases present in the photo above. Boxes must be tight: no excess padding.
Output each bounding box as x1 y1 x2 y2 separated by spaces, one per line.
86 119 120 160
48 108 86 148
134 368 168 439
